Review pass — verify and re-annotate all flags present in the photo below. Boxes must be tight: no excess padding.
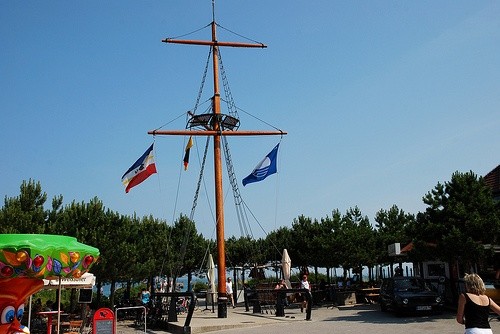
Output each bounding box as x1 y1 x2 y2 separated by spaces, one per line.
121 143 158 194
242 143 280 187
183 136 193 171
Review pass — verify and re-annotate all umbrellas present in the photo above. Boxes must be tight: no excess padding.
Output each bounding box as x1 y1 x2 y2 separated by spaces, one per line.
206 254 218 304
281 249 293 296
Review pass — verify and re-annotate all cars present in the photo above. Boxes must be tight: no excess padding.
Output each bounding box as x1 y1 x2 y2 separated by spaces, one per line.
380 276 445 316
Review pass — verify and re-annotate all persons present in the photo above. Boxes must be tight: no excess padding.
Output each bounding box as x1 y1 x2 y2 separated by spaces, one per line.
456 272 500 334
135 288 151 316
226 277 236 308
27 294 58 323
273 274 344 313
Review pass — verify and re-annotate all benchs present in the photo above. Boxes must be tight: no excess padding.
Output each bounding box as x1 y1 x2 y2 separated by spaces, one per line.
259 302 304 314
366 293 381 305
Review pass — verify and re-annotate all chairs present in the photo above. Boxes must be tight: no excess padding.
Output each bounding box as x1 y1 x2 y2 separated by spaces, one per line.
64 320 84 334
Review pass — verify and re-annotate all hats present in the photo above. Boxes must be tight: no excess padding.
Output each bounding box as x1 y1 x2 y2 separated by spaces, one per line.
303 275 307 278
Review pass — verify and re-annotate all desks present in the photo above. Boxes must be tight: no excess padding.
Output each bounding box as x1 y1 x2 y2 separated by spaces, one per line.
361 288 381 305
35 310 64 334
199 288 219 313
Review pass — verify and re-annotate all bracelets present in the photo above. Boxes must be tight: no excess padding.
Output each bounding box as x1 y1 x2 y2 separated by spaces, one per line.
463 320 464 324
